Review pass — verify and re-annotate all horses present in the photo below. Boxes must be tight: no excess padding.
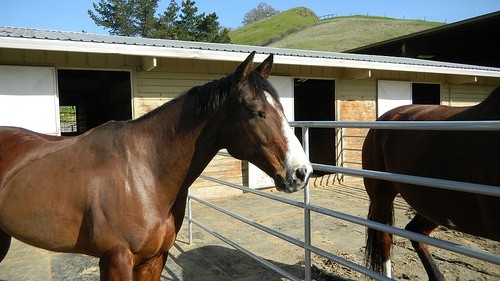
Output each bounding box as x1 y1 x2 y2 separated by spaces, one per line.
0 50 314 281
362 84 500 281
